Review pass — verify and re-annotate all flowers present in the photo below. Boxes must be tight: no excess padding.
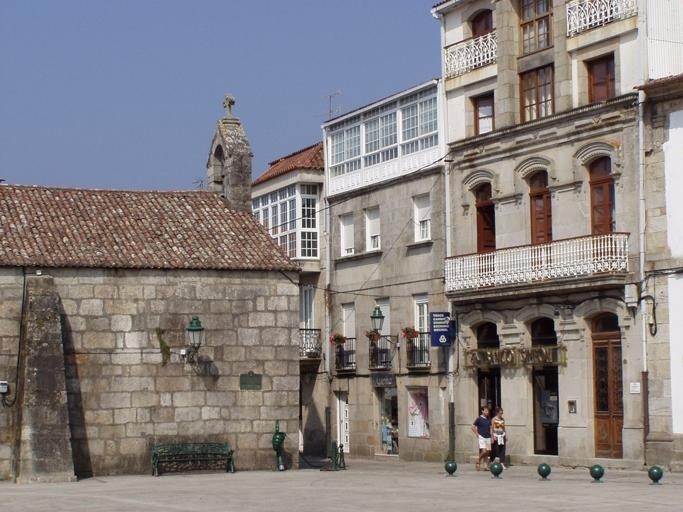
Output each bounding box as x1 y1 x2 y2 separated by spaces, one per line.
363 329 379 341
400 322 418 339
328 334 346 345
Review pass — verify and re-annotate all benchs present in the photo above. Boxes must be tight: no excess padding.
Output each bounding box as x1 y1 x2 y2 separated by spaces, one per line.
149 442 236 477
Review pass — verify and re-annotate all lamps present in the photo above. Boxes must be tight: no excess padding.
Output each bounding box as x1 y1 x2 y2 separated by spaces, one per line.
182 315 204 365
369 304 385 332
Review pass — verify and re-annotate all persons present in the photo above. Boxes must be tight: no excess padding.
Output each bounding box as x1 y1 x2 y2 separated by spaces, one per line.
468 406 493 471
489 407 507 471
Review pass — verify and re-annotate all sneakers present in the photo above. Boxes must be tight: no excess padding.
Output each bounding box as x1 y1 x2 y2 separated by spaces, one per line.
475 460 508 472
